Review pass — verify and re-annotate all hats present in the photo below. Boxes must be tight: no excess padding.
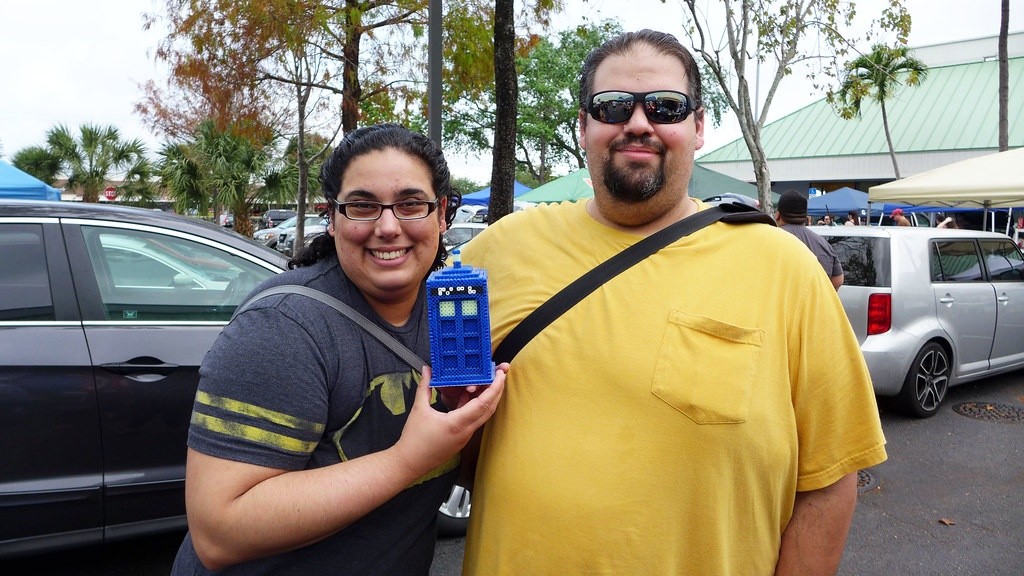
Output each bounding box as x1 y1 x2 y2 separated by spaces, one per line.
889 209 903 219
778 189 807 214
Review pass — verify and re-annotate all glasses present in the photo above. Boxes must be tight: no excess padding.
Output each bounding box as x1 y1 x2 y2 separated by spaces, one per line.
326 197 439 221
583 89 698 124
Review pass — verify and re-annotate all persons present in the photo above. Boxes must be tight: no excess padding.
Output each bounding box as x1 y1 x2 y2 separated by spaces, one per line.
935 214 959 230
1013 217 1024 250
890 209 911 226
776 190 844 292
175 123 509 576
817 210 865 226
429 29 890 575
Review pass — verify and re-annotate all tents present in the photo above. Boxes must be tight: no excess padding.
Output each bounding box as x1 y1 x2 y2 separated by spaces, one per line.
0 159 62 202
878 203 1024 217
865 144 1024 230
515 160 782 217
451 176 531 239
807 186 913 227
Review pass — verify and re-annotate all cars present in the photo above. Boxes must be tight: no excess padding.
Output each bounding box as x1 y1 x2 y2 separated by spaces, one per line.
0 202 488 576
805 224 1024 420
